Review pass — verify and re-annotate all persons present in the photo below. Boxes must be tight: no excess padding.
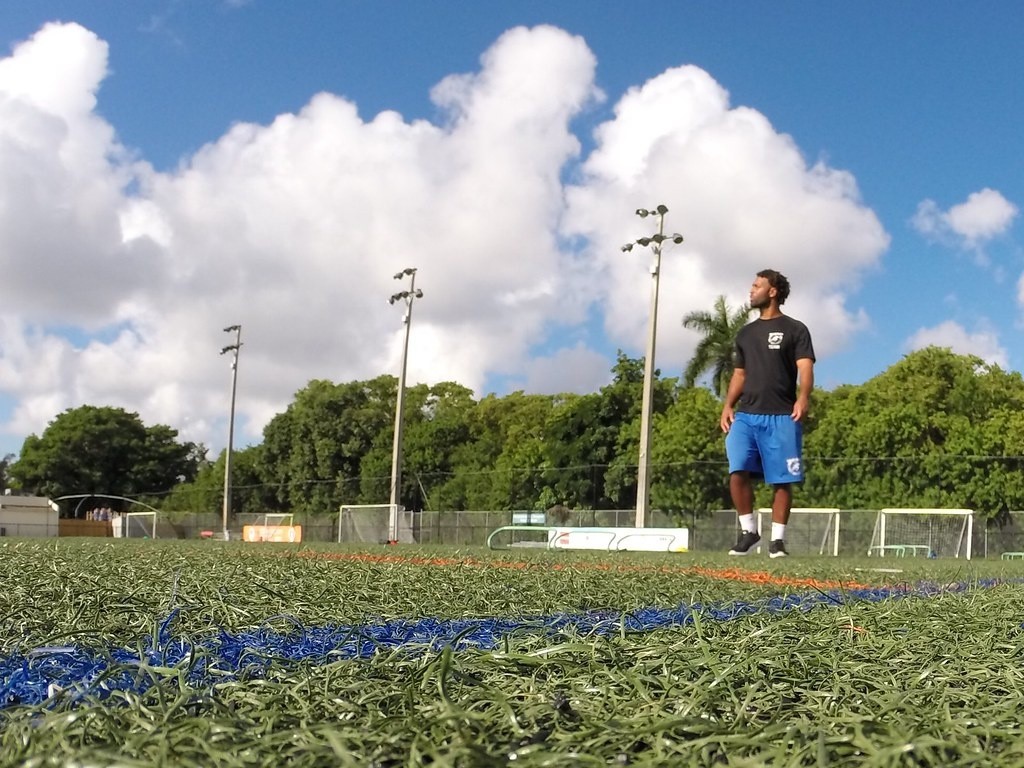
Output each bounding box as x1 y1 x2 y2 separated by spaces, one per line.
719 268 816 558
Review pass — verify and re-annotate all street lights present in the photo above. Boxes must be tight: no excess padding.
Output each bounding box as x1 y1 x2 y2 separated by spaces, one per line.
384 266 424 540
621 203 684 528
219 325 242 529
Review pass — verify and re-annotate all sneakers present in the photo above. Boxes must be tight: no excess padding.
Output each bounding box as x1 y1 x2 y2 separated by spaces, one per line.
728 529 761 555
768 539 789 559
